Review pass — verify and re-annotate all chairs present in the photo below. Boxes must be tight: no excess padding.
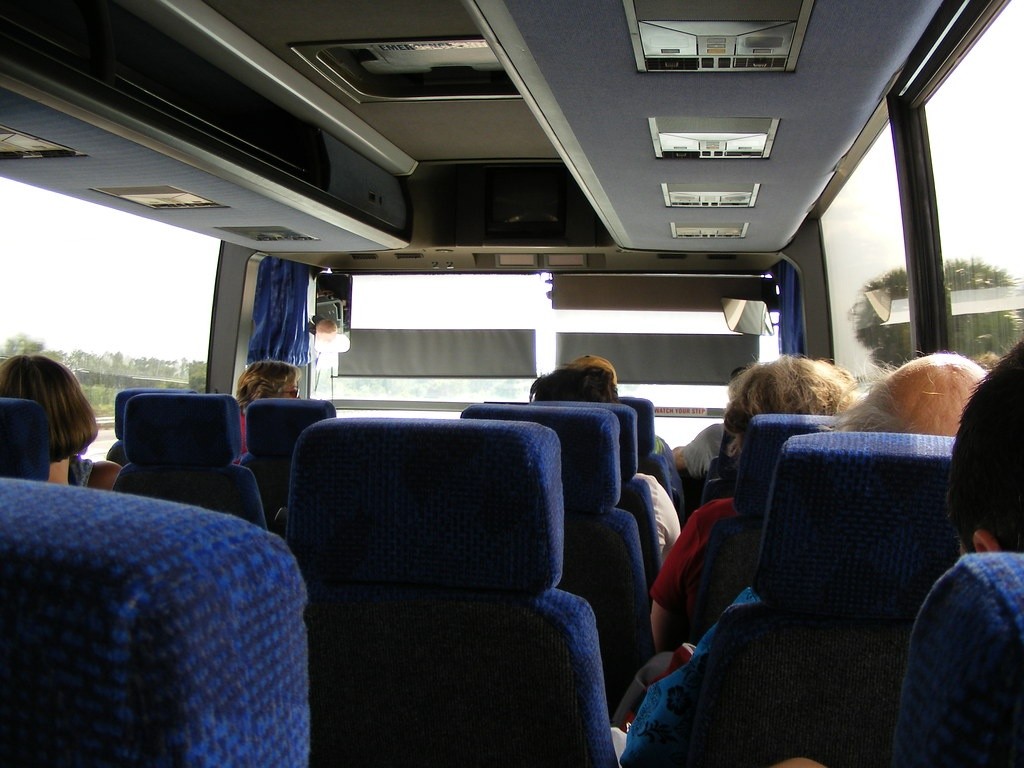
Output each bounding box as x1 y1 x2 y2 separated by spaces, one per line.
0 387 1024 768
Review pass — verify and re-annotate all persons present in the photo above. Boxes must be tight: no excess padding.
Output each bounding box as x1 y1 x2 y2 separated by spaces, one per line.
613 353 985 768
529 355 856 654
0 356 122 491
770 341 1024 768
236 361 302 464
